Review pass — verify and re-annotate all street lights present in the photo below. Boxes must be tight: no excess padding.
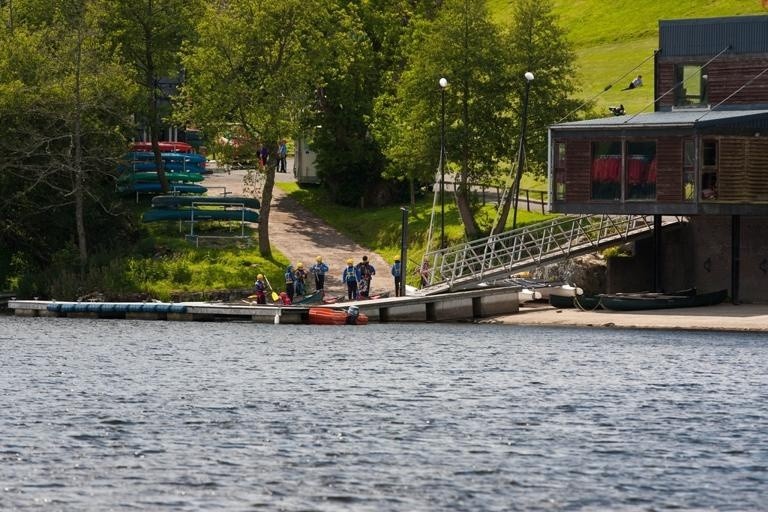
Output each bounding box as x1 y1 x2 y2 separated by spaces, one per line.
508 70 535 246
437 77 449 250
399 206 410 295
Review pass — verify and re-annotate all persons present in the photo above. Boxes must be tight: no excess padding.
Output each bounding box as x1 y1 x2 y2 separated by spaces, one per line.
256 139 288 173
619 75 643 91
608 104 625 115
254 254 430 304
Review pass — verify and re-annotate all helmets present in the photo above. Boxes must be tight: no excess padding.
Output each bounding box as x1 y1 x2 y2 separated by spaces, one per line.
394 255 401 261
257 256 352 280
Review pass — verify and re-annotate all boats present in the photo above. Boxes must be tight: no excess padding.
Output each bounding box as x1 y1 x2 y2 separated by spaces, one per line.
405 271 586 307
306 306 373 326
240 287 389 306
108 139 261 224
600 287 697 295
548 286 729 311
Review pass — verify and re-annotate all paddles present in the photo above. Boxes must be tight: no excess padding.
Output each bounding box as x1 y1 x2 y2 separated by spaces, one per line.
263 274 280 301
